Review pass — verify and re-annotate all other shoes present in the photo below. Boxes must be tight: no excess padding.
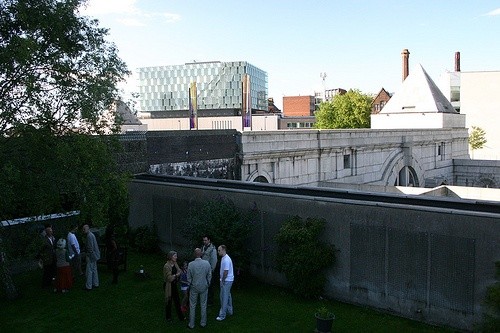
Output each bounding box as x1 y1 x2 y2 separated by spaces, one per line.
187 323 195 328
53 288 58 292
216 317 224 320
167 318 173 322
81 286 92 290
227 313 232 315
113 280 118 284
180 317 189 320
208 304 212 307
91 285 99 289
201 324 206 328
62 290 68 292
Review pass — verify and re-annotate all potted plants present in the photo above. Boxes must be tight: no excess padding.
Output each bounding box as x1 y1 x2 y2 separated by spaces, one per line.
314 297 335 332
136 264 145 276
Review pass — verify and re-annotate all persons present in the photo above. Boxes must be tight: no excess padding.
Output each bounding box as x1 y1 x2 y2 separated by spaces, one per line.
163 236 235 328
41 225 119 293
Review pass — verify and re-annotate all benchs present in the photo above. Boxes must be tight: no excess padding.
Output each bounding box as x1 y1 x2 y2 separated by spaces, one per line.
80 244 127 272
